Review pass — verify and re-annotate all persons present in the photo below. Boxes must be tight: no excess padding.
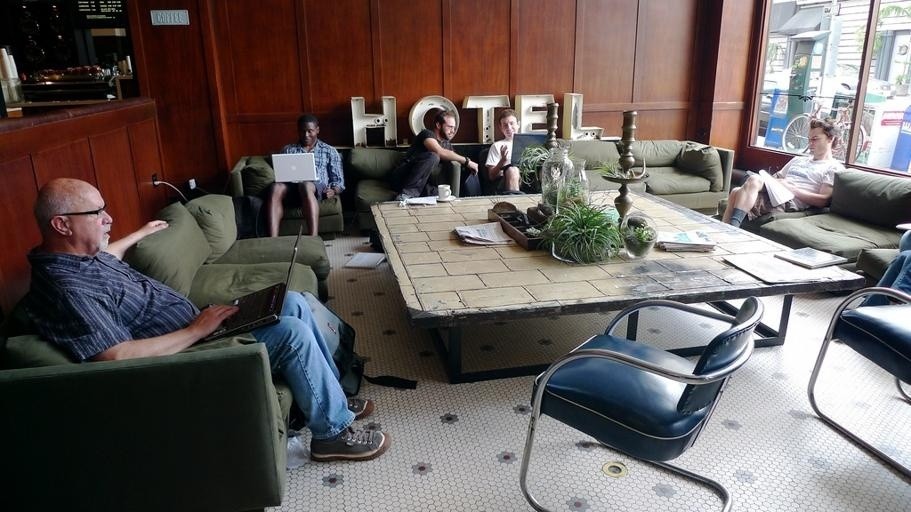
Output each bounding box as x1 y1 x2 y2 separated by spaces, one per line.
723 117 847 228
858 229 911 308
389 111 478 203
25 176 392 462
266 114 346 237
485 109 546 196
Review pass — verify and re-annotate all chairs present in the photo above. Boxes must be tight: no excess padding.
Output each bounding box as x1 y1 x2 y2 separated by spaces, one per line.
807 232 911 482
515 297 764 512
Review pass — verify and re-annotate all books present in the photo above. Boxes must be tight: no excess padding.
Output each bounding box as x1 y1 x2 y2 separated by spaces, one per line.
464 171 482 197
745 168 795 207
656 230 717 253
775 245 849 269
344 252 386 269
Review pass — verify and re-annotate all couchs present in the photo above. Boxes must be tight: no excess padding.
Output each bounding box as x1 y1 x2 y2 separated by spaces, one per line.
232 136 735 234
714 163 910 280
4 191 329 512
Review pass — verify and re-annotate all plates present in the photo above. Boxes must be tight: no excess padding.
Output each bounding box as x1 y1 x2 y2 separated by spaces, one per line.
436 195 455 202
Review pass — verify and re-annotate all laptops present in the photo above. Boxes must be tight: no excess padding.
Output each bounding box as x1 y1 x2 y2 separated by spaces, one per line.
500 133 546 169
202 225 303 341
271 152 320 183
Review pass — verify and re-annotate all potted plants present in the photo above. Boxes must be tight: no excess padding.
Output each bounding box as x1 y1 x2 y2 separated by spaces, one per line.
520 142 659 261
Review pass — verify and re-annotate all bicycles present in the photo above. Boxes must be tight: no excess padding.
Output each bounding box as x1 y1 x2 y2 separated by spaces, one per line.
780 90 871 165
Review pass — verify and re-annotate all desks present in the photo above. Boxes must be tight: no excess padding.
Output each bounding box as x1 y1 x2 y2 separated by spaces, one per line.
369 188 867 388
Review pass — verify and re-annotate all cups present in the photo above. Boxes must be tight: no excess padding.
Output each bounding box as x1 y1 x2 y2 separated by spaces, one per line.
438 185 452 199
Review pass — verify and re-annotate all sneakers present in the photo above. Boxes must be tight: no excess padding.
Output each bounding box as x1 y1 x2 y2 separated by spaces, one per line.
343 396 373 418
311 428 388 461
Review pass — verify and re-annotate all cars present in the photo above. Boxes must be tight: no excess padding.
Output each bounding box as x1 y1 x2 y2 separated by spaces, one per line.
789 61 897 104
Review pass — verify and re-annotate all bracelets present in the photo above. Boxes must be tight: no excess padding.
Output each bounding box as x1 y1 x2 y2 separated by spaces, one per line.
332 187 337 195
465 157 471 168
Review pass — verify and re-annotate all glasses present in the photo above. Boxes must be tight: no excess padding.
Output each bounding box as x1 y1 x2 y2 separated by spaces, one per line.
57 205 107 221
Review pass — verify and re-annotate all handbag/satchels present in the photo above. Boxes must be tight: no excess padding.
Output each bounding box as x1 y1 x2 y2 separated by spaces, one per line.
299 289 364 397
